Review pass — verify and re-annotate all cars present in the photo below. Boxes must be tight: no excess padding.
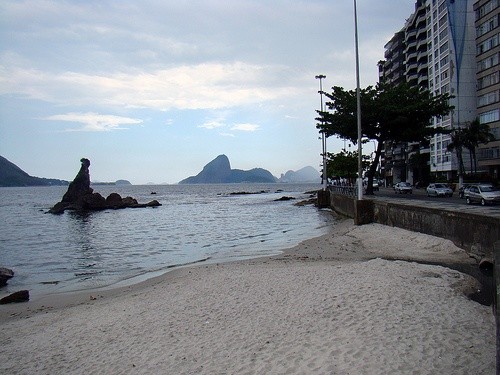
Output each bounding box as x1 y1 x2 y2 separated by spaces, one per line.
395 182 412 195
459 187 465 199
426 182 453 199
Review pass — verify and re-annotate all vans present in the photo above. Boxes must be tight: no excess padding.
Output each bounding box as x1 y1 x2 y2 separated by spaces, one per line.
463 184 500 206
364 178 379 192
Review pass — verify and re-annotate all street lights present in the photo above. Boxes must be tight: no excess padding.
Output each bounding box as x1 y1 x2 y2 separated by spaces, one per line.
314 73 328 192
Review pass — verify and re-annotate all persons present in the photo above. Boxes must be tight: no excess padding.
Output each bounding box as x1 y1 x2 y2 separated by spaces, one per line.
326 180 355 195
